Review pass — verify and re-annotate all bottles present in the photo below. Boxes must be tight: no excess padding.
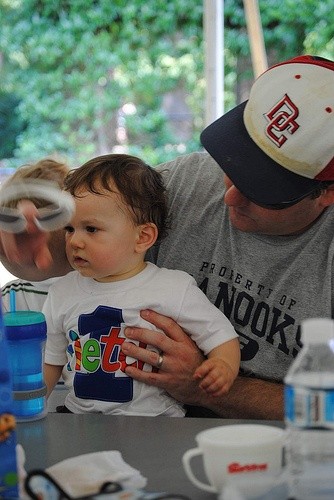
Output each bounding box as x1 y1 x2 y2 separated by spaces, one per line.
284 317 334 500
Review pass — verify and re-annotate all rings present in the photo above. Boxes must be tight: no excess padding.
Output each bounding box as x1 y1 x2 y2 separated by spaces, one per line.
156 355 164 369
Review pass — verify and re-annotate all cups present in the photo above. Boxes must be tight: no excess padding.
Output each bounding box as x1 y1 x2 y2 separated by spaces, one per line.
3 311 48 417
182 424 283 498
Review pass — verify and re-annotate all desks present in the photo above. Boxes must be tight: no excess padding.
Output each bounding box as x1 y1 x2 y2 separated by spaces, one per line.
0 412 290 500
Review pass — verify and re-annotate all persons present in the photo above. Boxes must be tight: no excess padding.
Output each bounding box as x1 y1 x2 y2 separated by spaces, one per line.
0 55 334 421
0 152 241 419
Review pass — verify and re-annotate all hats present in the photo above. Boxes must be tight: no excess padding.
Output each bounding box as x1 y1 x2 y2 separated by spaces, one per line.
199 55 334 210
